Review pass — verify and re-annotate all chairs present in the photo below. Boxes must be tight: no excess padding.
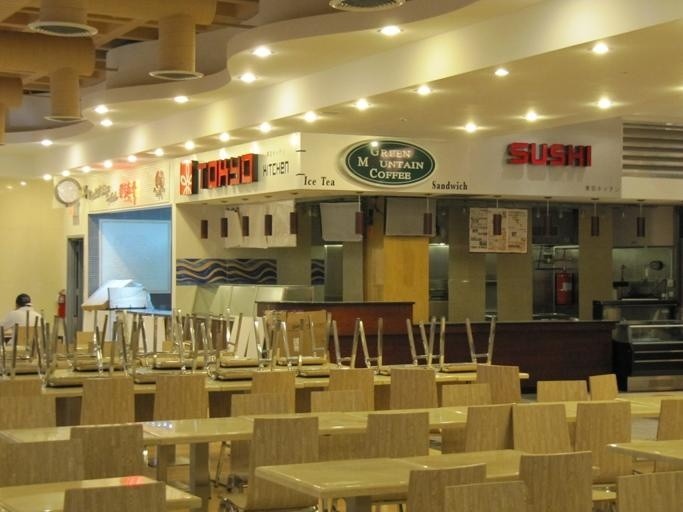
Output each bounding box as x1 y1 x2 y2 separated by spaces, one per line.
0 366 682 510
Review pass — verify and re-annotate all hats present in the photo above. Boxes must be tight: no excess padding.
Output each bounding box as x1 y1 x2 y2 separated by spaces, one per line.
16 294 31 306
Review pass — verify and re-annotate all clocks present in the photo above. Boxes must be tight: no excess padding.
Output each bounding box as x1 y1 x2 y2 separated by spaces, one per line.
53 176 81 207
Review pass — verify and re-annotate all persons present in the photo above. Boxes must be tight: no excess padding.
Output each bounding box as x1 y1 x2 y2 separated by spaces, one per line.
2 292 41 345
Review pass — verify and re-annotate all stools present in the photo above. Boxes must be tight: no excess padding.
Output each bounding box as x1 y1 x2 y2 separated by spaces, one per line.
0 367 683 510
0 301 500 390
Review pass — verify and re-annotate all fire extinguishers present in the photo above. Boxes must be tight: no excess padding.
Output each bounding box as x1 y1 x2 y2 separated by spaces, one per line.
58 289 65 318
